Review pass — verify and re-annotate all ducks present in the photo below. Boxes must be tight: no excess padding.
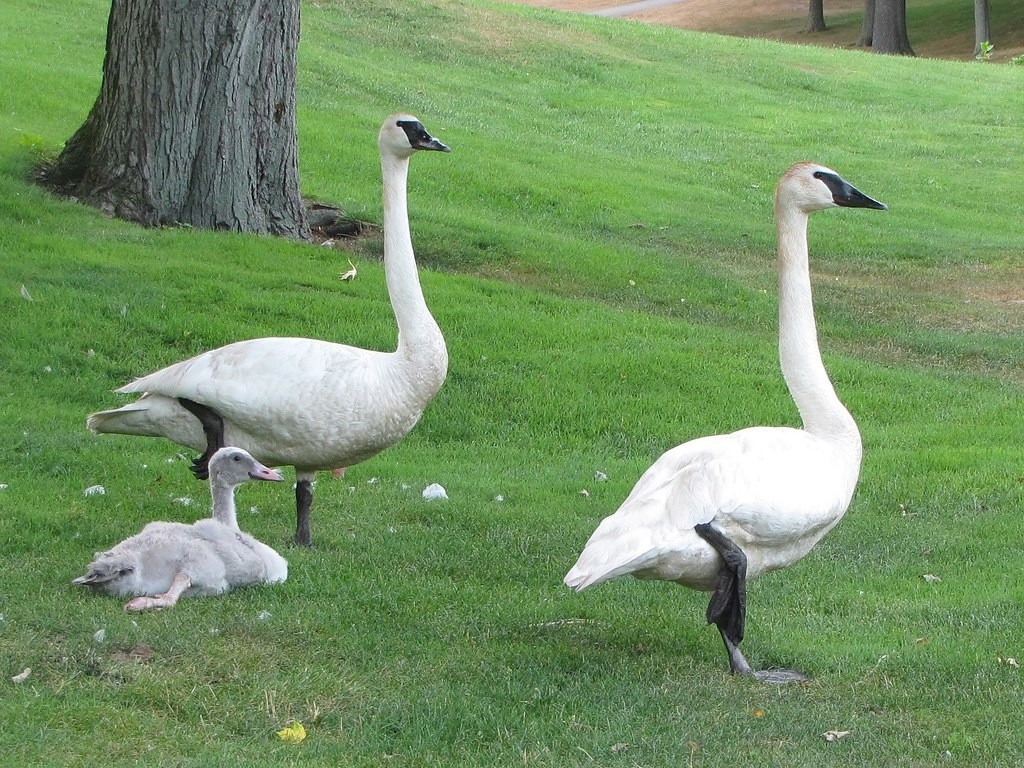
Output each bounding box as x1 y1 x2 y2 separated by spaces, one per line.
564 156 888 683
84 114 449 550
71 447 290 610
